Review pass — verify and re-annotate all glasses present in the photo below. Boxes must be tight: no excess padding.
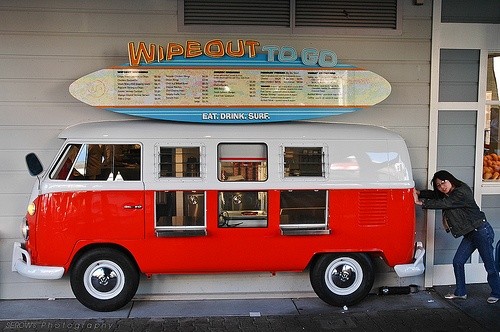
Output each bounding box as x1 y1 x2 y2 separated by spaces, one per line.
436 180 448 188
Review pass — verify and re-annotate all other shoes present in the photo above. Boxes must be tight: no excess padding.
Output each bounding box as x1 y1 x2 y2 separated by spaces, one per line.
487 297 499 303
445 293 467 299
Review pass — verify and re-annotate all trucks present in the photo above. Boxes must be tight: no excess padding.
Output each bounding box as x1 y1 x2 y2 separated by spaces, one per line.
11 118 426 312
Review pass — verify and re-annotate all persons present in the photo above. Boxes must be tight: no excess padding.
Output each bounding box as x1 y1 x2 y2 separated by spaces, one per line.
412 170 500 304
